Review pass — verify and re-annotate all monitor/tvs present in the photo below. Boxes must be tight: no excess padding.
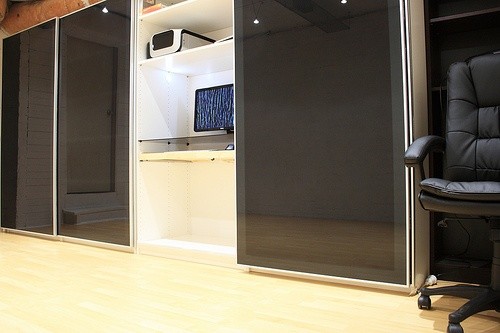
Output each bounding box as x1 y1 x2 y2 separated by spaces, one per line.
193 83 235 132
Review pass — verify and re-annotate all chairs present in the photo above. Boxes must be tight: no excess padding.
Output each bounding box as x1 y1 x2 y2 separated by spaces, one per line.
403 49 500 333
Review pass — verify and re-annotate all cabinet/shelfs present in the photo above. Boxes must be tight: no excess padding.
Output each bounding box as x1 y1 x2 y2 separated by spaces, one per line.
138 0 237 160
